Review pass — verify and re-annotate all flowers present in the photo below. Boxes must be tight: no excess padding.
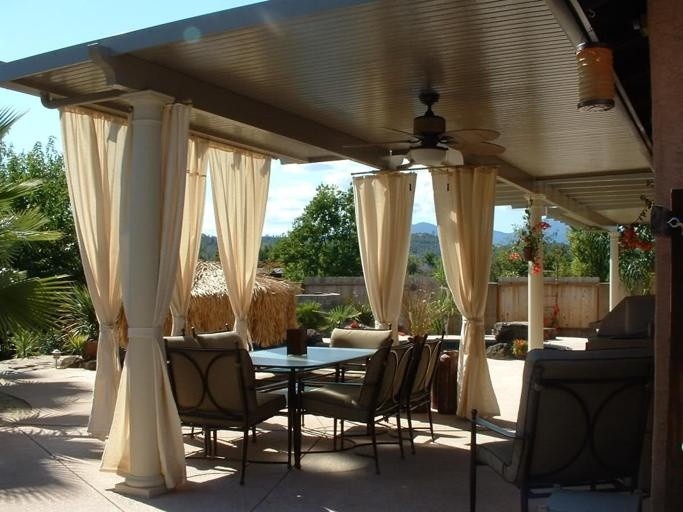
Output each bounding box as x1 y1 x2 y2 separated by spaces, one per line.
623 180 655 251
509 210 551 274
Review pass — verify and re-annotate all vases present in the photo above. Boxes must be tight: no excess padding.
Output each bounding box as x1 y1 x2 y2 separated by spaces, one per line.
524 246 533 261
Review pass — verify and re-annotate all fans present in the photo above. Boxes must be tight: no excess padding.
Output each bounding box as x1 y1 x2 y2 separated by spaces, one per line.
342 92 505 156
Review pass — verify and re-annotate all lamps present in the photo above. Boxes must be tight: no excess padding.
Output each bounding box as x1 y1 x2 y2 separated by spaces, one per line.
575 30 615 113
408 144 448 165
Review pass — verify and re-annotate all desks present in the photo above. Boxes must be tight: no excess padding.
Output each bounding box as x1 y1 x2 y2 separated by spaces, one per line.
242 340 376 471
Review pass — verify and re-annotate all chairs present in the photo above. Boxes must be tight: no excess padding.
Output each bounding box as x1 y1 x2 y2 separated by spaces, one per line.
471 347 654 512
165 327 287 483
300 330 445 474
586 295 655 351
328 325 393 374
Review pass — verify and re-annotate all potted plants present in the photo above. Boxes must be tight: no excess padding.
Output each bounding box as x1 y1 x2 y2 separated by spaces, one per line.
57 284 99 358
